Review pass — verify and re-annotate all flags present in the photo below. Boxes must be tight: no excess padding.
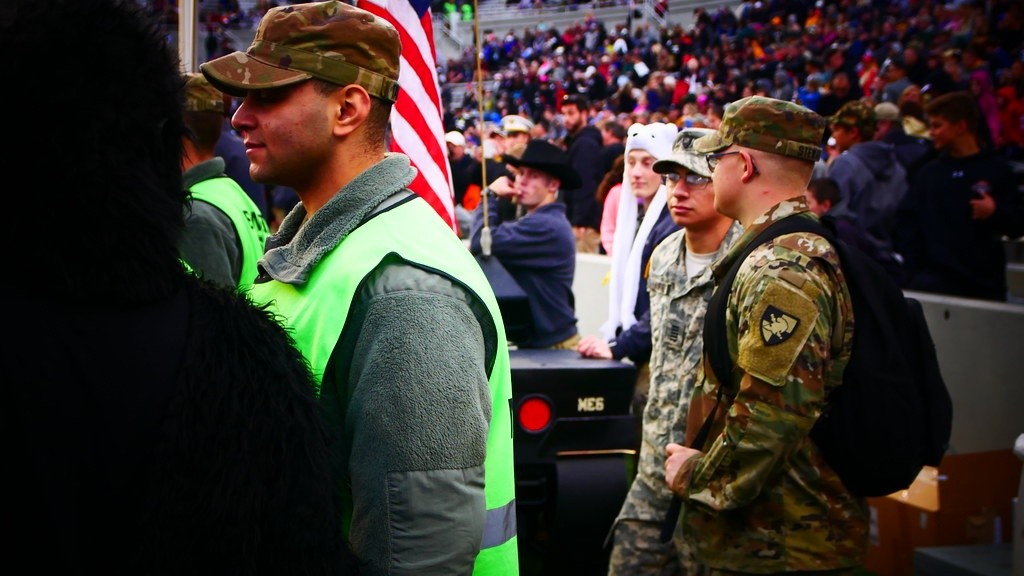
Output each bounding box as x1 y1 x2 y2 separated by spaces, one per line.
354 0 459 236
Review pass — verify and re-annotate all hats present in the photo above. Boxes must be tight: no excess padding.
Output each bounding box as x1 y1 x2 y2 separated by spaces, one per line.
500 115 534 138
602 122 678 340
651 127 718 179
183 67 225 108
445 131 465 147
199 0 403 104
828 102 879 133
561 94 587 111
693 96 824 162
514 139 572 184
648 36 660 48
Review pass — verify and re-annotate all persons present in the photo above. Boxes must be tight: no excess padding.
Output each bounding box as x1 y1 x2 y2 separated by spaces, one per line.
197 1 301 233
201 0 520 576
1 0 328 575
606 127 742 576
432 0 1024 414
179 72 271 302
664 96 870 576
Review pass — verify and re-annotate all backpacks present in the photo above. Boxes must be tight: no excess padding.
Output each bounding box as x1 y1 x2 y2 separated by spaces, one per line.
703 211 954 500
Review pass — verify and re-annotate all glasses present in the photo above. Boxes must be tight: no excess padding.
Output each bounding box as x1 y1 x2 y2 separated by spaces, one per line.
833 119 855 135
704 149 741 173
658 171 710 190
